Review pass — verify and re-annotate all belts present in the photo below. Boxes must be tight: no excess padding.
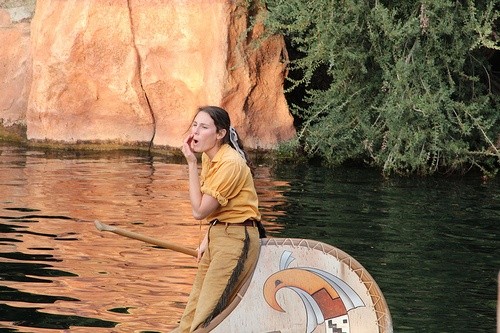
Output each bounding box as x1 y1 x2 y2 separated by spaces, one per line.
212 219 254 226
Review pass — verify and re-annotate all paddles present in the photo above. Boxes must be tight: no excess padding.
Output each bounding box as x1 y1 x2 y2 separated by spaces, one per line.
93 218 197 257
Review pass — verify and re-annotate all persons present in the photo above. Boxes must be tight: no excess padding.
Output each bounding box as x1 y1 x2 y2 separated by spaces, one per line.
177 106 260 333
